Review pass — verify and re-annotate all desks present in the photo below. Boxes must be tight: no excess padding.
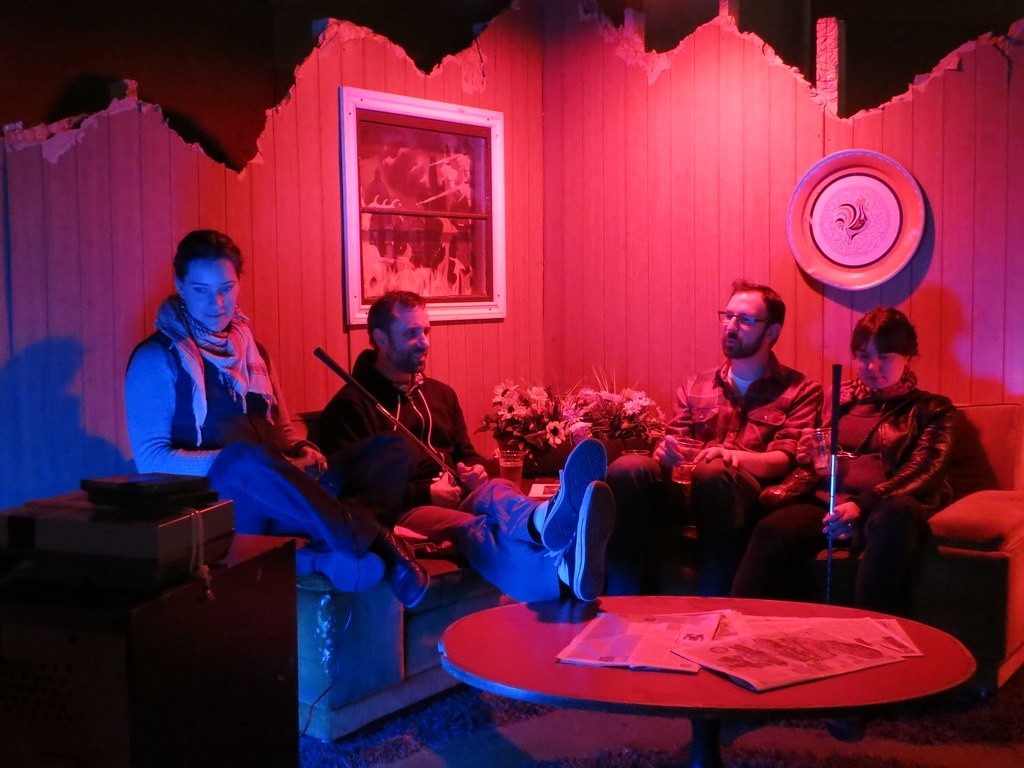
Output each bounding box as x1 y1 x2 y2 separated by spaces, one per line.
440 594 977 768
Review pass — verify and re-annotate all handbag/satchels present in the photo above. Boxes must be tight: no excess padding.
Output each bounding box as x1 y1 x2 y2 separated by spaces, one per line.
839 452 889 492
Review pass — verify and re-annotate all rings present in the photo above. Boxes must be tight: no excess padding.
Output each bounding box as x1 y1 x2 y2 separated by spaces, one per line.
847 524 851 526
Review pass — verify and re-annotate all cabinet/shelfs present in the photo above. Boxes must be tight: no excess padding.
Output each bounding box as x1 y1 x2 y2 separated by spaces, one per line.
0 475 296 768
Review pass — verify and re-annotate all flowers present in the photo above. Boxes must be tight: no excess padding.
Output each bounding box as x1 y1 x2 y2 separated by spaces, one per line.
473 370 665 454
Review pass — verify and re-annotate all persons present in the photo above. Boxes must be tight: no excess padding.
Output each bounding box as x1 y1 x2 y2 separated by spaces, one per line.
604 285 825 597
314 289 616 603
125 229 431 609
730 304 959 615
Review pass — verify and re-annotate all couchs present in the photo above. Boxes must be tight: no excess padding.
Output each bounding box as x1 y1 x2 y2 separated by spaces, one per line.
682 401 1024 689
291 411 495 743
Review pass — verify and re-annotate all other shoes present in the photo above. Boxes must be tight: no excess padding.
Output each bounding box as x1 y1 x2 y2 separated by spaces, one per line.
563 480 617 604
823 712 864 742
367 525 429 608
292 544 385 592
541 439 608 552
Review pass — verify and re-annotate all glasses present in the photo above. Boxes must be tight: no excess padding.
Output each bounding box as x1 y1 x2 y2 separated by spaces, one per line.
723 310 770 331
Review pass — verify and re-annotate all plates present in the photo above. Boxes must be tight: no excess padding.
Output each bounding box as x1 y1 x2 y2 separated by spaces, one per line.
786 150 925 291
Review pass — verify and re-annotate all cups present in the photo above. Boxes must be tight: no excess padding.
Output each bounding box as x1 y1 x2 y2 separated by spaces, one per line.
807 427 831 474
671 438 704 485
622 450 650 458
500 450 524 488
